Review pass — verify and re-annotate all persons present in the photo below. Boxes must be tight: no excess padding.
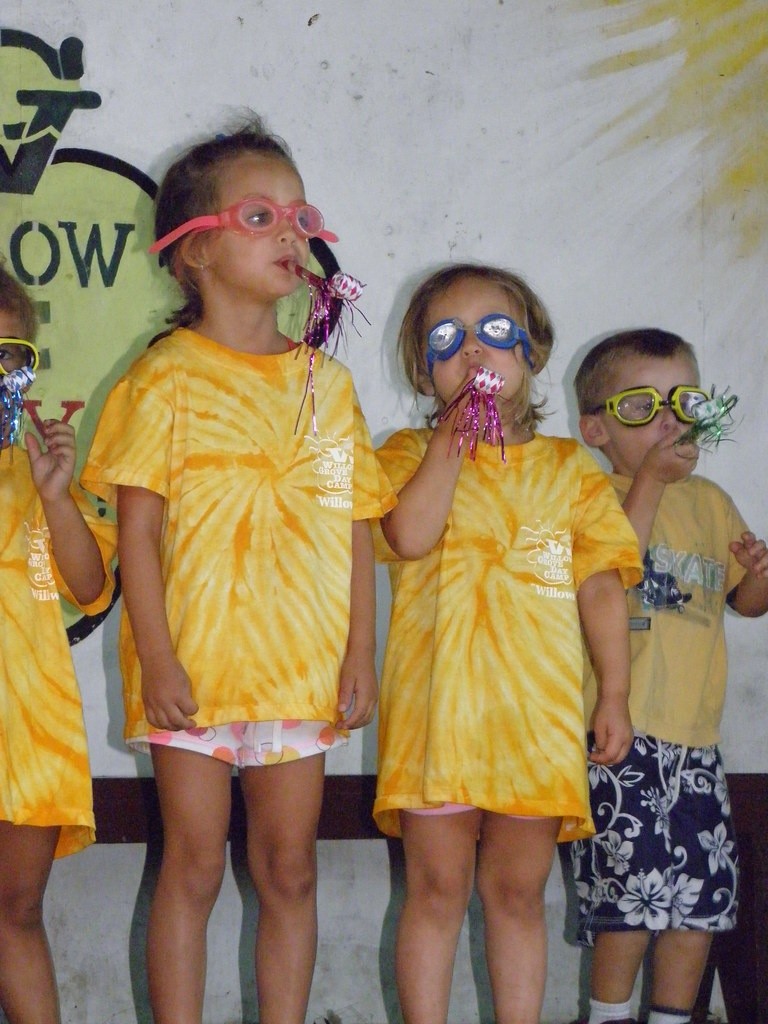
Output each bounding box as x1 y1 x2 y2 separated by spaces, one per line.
370 257 648 1024
0 261 123 1024
567 328 768 1024
77 99 398 1024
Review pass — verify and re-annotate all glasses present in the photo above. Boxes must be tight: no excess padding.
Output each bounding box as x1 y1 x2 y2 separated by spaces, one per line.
0 337 39 380
427 313 537 378
580 385 714 427
148 197 340 254
